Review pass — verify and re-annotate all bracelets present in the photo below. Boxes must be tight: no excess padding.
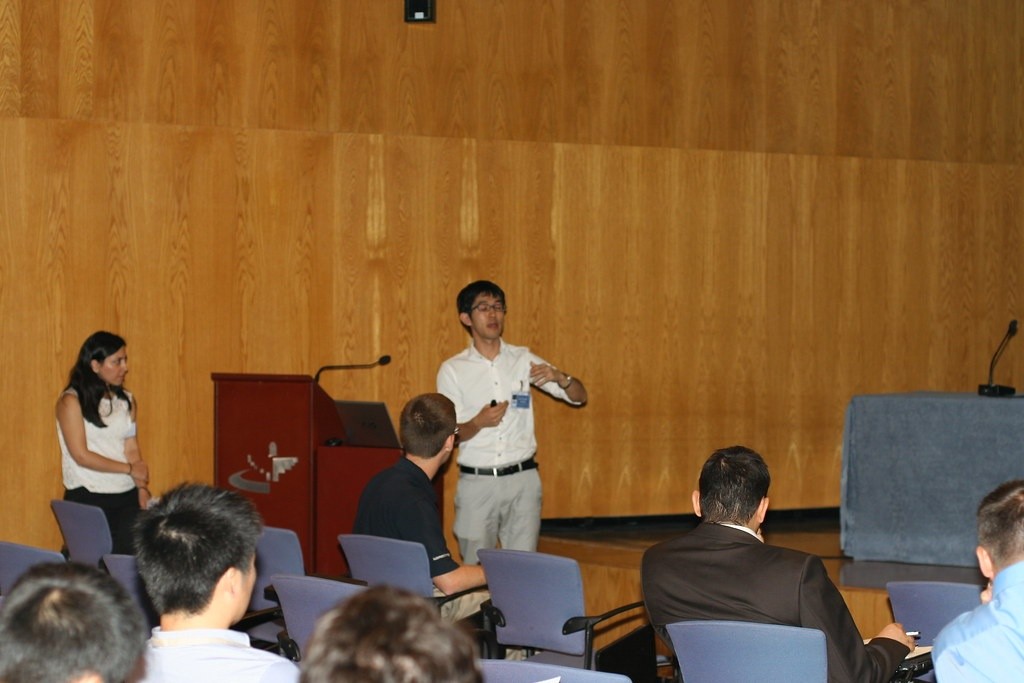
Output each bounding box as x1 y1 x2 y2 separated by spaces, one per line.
127 463 133 475
139 486 148 490
558 373 573 389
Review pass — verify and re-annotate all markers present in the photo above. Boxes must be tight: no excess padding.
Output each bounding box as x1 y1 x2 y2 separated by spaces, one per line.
491 400 497 407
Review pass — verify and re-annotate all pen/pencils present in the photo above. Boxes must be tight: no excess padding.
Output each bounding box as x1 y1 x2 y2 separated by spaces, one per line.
905 631 921 636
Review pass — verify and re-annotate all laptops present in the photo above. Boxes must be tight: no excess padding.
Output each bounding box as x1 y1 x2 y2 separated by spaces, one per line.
333 399 405 449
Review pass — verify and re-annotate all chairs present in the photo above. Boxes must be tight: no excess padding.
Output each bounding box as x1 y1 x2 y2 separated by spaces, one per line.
0 499 988 683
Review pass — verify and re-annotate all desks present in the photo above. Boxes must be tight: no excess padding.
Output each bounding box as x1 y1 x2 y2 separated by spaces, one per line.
838 391 1024 567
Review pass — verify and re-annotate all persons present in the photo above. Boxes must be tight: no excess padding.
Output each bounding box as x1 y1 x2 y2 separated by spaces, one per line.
300 586 487 683
132 480 306 683
639 446 916 683
0 560 146 683
435 278 590 565
351 392 528 662
53 328 153 557
930 479 1024 683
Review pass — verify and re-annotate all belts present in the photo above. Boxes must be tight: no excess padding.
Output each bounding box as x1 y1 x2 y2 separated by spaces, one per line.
460 456 540 477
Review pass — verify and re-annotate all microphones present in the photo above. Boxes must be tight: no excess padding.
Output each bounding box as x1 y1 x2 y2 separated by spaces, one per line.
978 319 1018 395
313 355 391 382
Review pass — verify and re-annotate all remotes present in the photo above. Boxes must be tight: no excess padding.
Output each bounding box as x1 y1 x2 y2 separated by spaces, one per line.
490 400 497 407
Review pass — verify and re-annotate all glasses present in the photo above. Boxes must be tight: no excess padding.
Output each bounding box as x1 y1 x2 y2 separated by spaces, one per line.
466 302 507 312
